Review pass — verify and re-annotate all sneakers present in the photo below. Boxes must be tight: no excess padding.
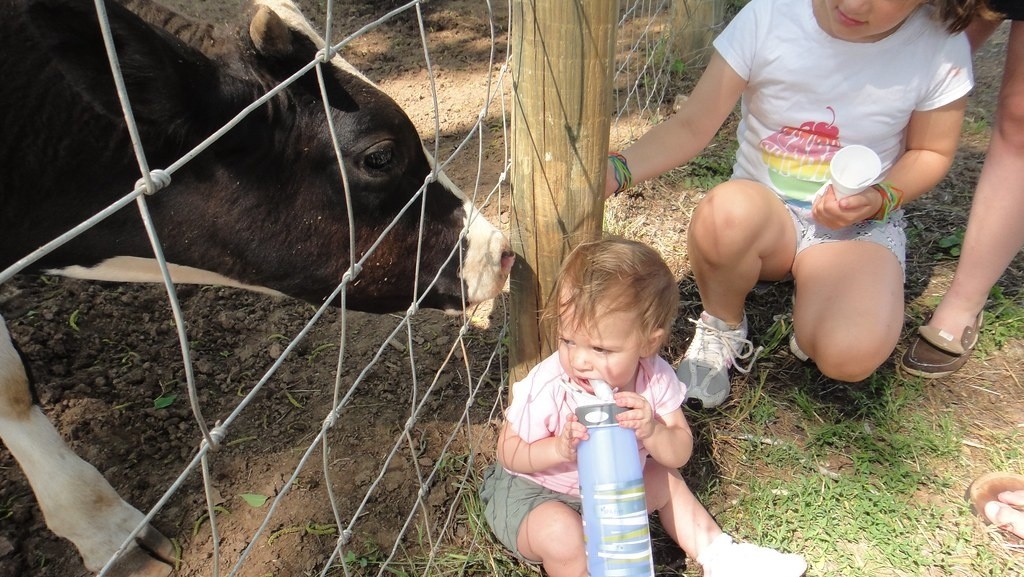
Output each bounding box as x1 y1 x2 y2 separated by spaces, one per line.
677 307 764 409
789 337 810 361
703 541 807 577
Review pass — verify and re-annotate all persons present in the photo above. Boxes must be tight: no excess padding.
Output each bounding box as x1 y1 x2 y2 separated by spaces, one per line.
983 488 1024 540
900 0 1024 380
480 235 808 577
603 0 978 411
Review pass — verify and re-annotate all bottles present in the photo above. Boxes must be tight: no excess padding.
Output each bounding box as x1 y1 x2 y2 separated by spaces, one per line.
575 379 655 577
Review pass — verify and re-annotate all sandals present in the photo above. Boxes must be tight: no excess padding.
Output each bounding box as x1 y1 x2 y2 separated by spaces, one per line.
970 471 1024 544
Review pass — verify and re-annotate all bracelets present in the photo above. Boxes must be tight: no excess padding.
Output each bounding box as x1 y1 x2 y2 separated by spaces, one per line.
863 181 904 224
607 151 633 197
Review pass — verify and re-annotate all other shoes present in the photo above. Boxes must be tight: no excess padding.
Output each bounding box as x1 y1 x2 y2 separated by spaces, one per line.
900 304 985 378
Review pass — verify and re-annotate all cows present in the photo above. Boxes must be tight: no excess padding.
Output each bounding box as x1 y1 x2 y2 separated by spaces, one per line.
0 0 513 576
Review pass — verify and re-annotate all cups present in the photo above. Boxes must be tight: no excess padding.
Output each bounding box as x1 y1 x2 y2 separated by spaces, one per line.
830 145 882 201
